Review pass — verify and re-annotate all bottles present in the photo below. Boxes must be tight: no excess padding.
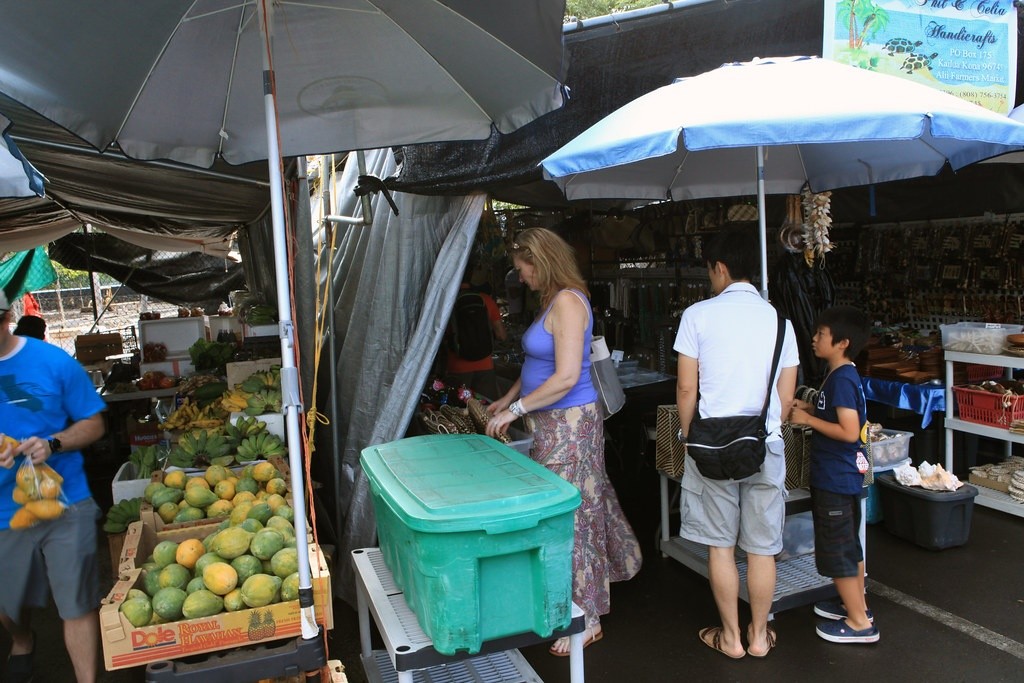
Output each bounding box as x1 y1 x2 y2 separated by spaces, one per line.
217 329 236 342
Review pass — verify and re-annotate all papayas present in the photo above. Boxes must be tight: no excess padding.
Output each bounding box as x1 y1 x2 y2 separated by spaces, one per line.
119 462 301 630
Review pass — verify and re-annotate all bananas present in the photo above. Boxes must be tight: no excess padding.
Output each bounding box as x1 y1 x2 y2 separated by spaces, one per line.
129 365 283 479
103 497 141 533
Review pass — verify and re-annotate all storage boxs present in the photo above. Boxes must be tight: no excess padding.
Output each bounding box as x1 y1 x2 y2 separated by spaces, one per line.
969 472 1009 493
507 427 536 459
359 432 583 656
952 363 1024 430
75 316 334 671
939 321 1024 355
866 414 978 552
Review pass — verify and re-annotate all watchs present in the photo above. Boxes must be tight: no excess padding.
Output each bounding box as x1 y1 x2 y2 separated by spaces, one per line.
509 403 523 417
46 436 61 452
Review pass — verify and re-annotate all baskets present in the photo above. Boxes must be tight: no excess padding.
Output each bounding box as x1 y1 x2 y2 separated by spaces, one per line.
952 378 1023 430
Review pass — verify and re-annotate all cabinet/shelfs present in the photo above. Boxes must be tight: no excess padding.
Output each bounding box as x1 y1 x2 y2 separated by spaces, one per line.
943 350 1024 517
656 470 870 629
350 547 585 683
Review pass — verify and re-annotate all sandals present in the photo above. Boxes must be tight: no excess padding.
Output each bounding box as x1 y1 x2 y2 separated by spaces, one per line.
818 618 879 644
549 626 604 655
814 601 874 620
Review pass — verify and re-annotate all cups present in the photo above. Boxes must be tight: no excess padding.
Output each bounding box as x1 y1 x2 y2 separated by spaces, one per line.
87 371 105 388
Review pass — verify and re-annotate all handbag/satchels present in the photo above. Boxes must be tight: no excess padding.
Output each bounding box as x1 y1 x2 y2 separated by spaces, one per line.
656 405 683 476
589 336 626 420
792 385 874 488
686 413 767 480
782 424 799 490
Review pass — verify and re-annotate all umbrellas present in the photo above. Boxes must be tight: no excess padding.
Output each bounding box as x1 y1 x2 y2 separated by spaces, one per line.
536 54 1024 300
0 0 567 640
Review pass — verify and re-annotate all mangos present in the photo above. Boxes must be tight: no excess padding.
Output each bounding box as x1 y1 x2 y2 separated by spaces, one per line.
8 462 65 528
0 435 22 458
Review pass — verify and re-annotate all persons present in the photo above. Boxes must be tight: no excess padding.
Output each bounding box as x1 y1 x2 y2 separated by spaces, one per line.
139 304 232 338
504 268 526 323
438 258 508 401
788 305 880 643
486 227 643 656
671 231 801 658
13 315 45 342
0 284 106 683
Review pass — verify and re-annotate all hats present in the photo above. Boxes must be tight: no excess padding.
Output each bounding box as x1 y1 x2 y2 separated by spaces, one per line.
0 288 11 315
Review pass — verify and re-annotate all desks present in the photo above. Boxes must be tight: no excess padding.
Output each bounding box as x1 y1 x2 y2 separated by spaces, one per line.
102 386 179 401
858 376 956 467
602 373 677 530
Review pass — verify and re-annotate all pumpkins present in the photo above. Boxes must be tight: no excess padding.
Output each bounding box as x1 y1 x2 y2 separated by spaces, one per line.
159 377 173 388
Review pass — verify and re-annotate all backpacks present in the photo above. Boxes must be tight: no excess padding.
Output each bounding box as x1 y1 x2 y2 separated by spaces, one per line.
450 288 496 363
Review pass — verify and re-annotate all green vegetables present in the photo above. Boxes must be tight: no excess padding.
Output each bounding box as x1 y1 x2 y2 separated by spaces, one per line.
244 306 277 326
187 339 235 375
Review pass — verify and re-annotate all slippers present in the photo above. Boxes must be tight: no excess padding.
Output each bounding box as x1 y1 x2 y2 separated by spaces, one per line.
747 629 777 657
699 626 745 658
